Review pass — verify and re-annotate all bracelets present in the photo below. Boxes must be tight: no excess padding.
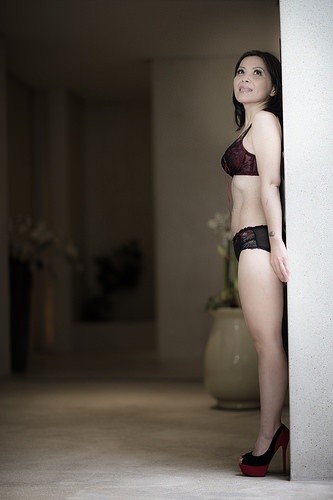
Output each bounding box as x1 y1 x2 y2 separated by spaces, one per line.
268 231 282 238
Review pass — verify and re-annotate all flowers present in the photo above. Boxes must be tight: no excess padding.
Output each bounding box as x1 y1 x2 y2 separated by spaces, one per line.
206 212 241 310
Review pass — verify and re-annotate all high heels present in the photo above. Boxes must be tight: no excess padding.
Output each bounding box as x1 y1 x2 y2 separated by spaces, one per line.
239 424 289 476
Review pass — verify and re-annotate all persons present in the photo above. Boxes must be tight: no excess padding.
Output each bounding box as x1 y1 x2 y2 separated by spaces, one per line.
220 51 290 476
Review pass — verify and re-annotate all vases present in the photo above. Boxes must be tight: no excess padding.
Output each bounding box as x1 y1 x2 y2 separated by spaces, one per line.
204 307 260 409
